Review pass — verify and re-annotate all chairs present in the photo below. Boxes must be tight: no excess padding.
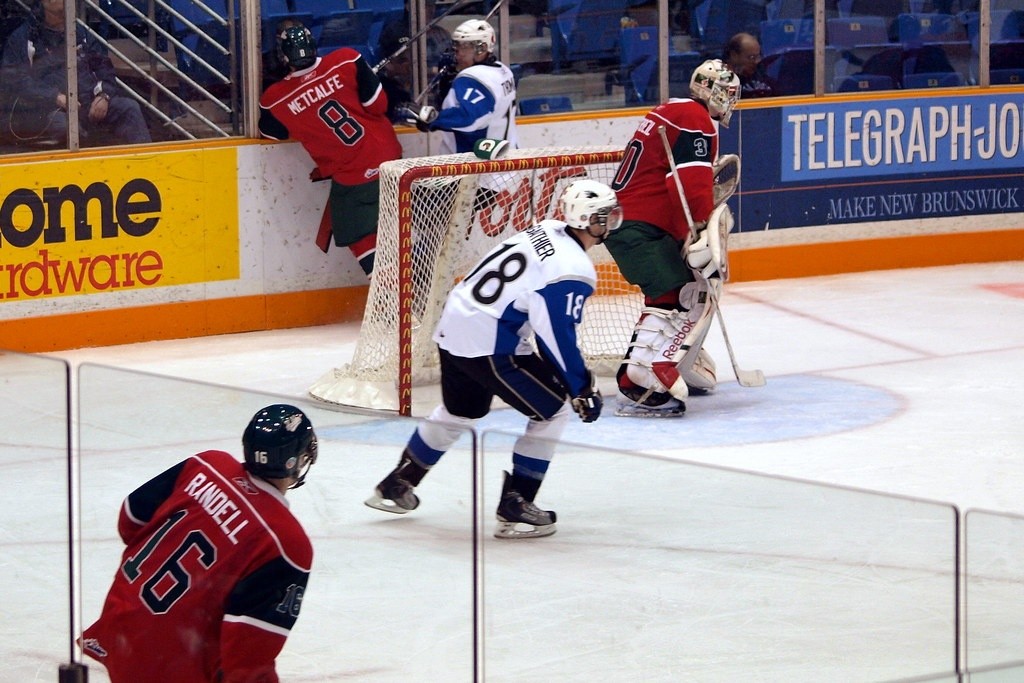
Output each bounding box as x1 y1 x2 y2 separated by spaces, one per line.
549 0 1024 101
100 0 520 110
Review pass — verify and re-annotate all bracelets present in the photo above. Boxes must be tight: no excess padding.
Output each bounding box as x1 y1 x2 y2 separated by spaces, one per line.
97 92 111 101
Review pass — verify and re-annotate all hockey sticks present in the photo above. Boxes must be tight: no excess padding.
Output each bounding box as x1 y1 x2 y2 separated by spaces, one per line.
414 0 504 106
657 125 768 389
371 0 475 73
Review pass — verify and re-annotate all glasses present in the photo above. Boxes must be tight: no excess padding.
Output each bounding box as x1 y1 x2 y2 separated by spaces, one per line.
750 55 761 60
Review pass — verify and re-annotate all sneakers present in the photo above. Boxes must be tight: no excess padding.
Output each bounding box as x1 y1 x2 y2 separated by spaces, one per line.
364 449 428 514
494 470 557 538
615 389 686 416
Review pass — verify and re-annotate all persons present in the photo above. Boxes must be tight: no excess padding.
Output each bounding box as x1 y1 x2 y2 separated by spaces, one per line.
688 0 768 58
720 32 778 99
783 49 866 95
77 404 319 683
374 180 624 526
603 59 741 412
0 0 153 149
256 0 516 333
861 10 1024 90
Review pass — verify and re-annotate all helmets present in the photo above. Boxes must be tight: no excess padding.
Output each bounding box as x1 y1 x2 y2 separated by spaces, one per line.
690 59 741 128
243 404 317 479
451 19 496 57
281 26 316 68
560 180 618 229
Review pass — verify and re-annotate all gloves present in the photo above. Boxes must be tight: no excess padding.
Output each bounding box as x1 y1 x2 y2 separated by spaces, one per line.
394 102 438 122
571 369 602 424
